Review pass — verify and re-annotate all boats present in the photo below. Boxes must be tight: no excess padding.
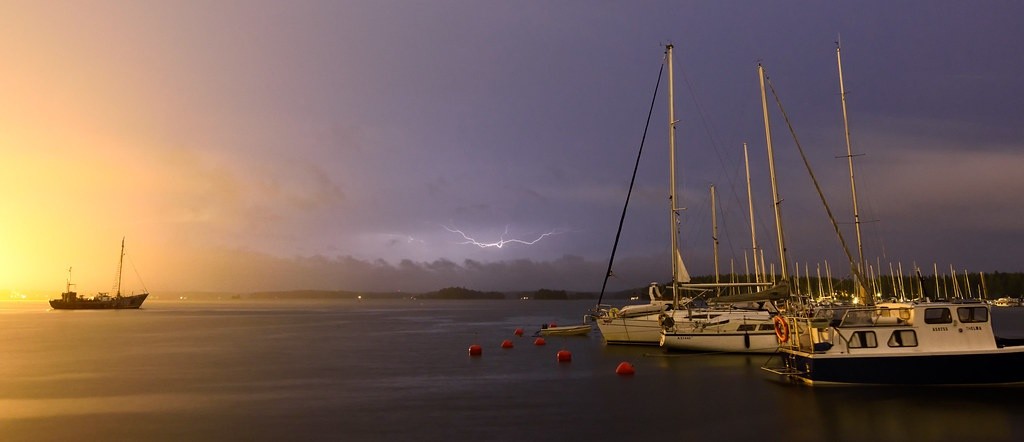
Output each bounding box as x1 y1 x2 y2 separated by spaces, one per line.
541 326 591 335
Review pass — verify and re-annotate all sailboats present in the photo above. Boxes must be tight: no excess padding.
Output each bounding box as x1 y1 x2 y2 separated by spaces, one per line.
763 47 1024 387
594 38 760 346
657 43 870 357
49 236 149 310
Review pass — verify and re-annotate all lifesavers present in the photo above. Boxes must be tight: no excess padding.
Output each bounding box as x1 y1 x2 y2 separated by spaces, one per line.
774 315 788 342
609 307 619 318
